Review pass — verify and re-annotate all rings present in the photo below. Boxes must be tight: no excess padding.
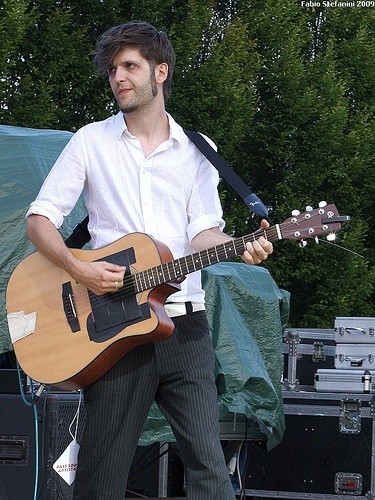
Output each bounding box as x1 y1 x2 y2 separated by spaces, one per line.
114 282 118 288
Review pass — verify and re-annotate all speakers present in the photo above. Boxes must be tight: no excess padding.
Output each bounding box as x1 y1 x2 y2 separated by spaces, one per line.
0 392 86 500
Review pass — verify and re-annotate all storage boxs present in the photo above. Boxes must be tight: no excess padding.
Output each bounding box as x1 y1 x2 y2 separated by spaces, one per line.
125 316 375 500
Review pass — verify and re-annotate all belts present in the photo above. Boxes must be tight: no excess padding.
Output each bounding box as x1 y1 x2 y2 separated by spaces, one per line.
163 300 206 317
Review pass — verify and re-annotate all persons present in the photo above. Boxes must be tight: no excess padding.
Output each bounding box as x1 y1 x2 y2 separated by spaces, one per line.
24 20 275 500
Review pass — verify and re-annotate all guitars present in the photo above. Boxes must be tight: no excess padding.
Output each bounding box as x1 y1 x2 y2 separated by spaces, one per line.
5 200 350 392
